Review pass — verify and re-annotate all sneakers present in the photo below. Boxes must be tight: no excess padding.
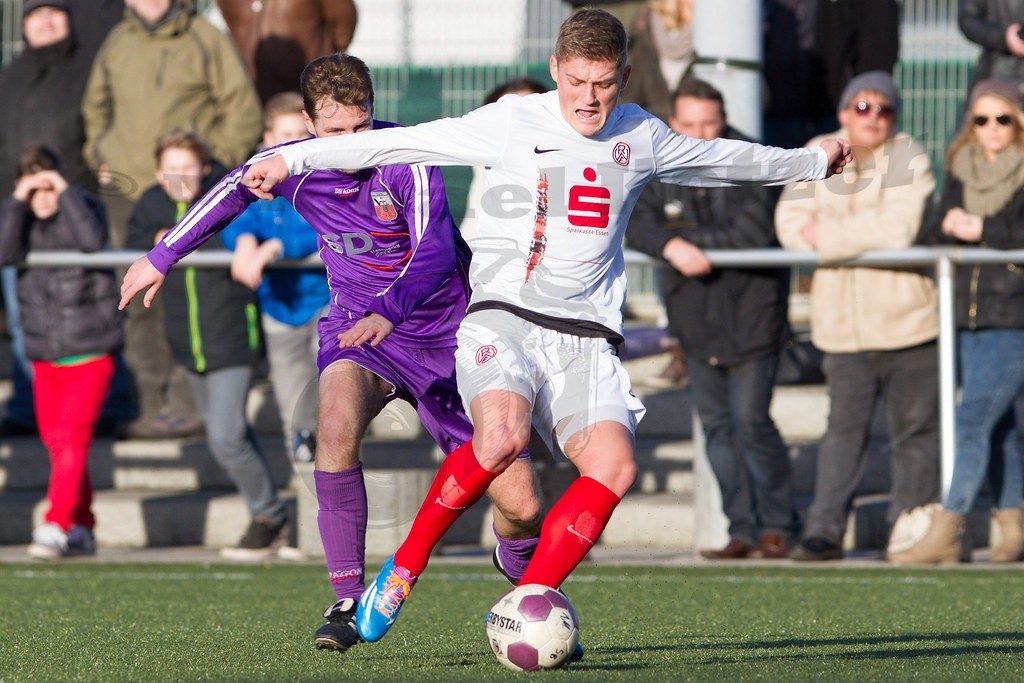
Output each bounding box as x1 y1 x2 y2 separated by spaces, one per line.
355 553 418 643
492 543 524 588
312 597 365 652
484 609 584 662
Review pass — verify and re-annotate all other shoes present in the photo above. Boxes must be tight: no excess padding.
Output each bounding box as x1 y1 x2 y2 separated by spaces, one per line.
802 537 842 560
25 519 68 562
237 515 291 550
61 526 97 554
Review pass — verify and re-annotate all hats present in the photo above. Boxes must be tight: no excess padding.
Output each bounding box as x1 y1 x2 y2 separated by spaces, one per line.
969 78 1024 108
840 74 901 110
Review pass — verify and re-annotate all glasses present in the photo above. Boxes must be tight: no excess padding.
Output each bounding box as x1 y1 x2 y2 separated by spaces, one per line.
853 101 895 119
971 114 1014 128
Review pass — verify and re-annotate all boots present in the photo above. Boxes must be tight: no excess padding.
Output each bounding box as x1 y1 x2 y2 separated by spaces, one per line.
991 507 1024 562
886 509 965 565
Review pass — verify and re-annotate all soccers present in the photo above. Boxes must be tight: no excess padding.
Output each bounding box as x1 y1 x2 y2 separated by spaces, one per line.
485 583 580 673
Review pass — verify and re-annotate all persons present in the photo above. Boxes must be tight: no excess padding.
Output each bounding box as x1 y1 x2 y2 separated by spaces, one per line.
242 10 939 644
0 0 358 561
757 0 1024 568
119 49 566 651
568 0 695 126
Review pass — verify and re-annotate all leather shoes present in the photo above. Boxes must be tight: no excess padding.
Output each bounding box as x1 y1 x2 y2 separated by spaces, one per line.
699 539 756 559
760 532 787 556
